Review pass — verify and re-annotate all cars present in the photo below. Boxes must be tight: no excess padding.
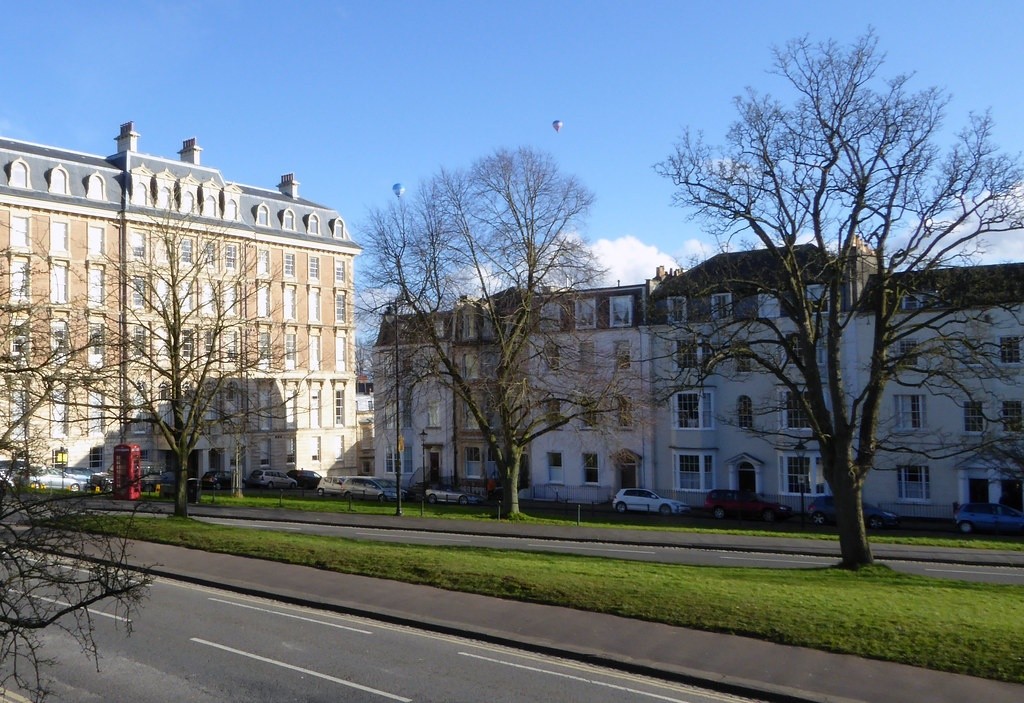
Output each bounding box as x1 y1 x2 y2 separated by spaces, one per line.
342 478 406 506
286 469 323 489
200 470 245 491
21 464 83 492
58 466 97 488
612 488 690 515
426 485 480 504
953 502 1024 534
249 469 297 490
704 488 793 522
0 460 30 488
316 477 346 497
805 495 898 528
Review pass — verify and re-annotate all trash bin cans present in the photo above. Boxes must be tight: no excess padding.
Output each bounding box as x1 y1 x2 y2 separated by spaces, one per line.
186 478 202 503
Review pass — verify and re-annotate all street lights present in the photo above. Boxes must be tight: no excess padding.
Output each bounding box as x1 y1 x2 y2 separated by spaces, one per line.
378 299 402 516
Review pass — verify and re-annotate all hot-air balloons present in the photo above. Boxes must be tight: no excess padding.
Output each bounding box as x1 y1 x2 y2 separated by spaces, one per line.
392 183 406 200
553 120 563 134
